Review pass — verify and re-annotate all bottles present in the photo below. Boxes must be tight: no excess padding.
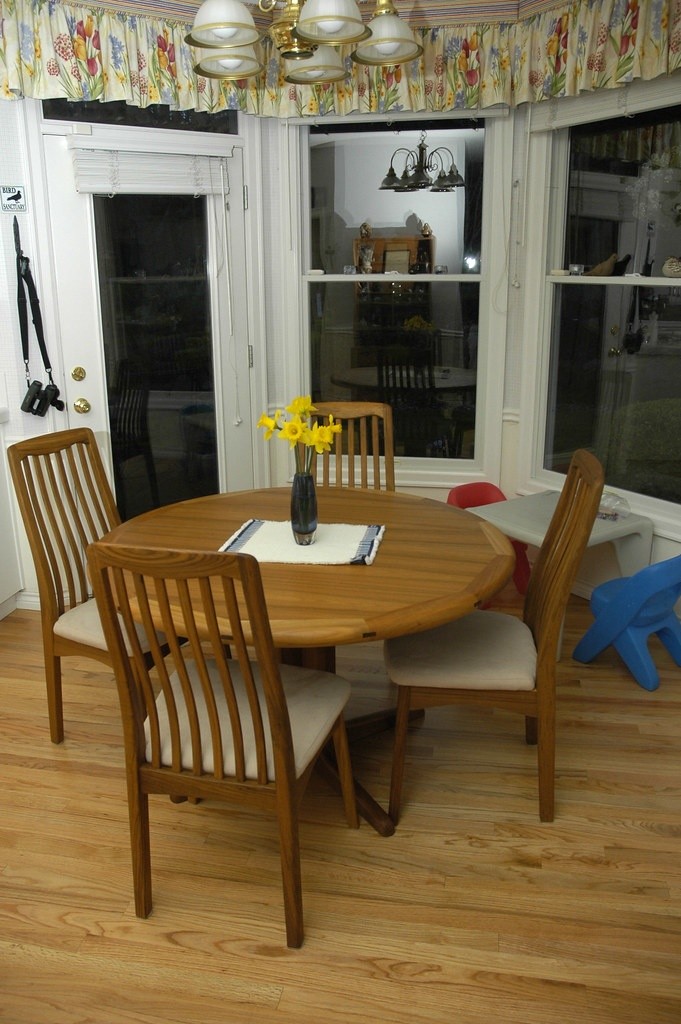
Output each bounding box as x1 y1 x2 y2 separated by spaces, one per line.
649 310 659 344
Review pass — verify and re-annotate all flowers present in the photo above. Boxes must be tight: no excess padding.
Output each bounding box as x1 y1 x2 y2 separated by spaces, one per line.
648 257 681 312
259 393 344 531
404 313 436 338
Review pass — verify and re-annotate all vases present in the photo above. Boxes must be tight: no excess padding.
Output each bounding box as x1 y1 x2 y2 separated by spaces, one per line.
648 311 658 344
407 333 431 373
290 472 318 546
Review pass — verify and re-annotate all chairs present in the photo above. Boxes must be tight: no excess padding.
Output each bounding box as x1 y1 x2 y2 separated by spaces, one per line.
9 428 187 746
85 541 357 951
300 400 394 492
572 553 681 691
109 358 161 521
371 330 477 457
445 481 530 610
388 448 603 830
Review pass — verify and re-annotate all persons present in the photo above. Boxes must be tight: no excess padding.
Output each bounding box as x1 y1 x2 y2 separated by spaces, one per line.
354 223 374 293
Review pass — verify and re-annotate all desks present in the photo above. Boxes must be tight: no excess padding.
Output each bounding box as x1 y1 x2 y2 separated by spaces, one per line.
93 486 516 837
332 364 478 458
462 490 654 578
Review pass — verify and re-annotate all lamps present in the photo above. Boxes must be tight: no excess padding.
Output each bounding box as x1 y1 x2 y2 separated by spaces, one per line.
378 129 466 193
184 0 424 84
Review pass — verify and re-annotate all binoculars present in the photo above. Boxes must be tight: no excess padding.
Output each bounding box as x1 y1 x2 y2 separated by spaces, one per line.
21 380 57 417
